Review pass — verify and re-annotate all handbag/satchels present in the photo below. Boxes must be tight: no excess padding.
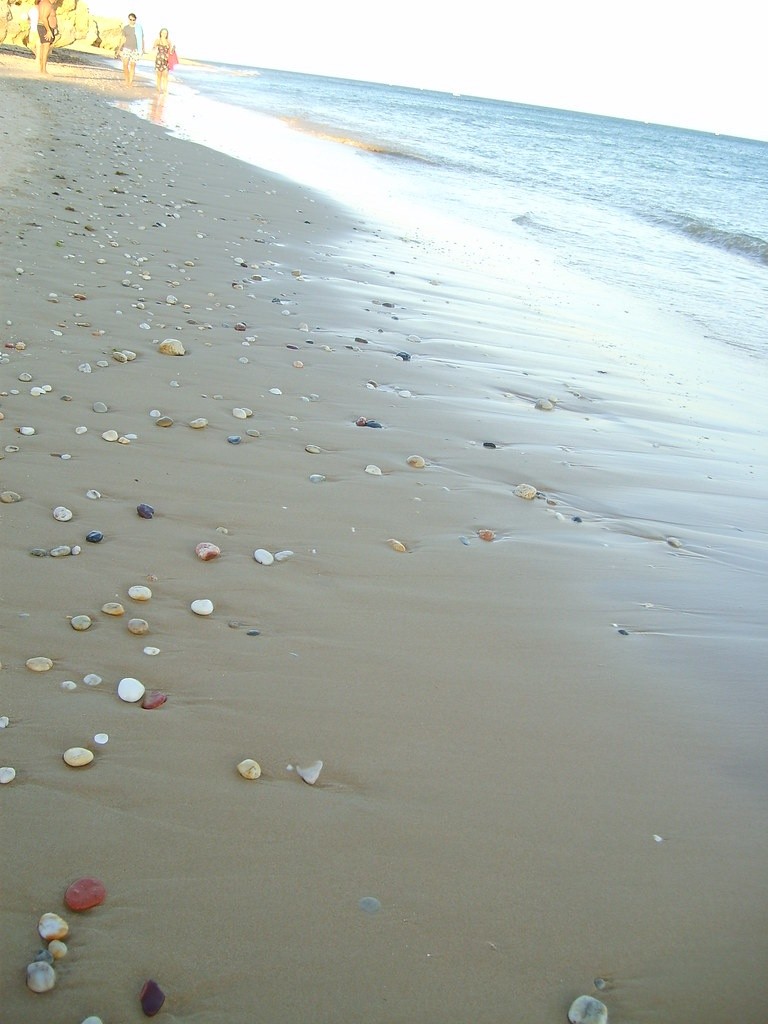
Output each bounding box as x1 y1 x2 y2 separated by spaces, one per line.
167 43 178 70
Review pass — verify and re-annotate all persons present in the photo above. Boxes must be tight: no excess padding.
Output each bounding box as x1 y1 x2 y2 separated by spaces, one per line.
152 28 176 95
28 0 45 72
36 0 64 77
116 13 144 86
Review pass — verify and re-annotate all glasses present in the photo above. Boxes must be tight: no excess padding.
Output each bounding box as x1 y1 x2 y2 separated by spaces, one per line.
130 19 136 21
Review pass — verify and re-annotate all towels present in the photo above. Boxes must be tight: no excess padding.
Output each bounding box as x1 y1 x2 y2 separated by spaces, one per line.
168 50 179 70
134 23 142 53
